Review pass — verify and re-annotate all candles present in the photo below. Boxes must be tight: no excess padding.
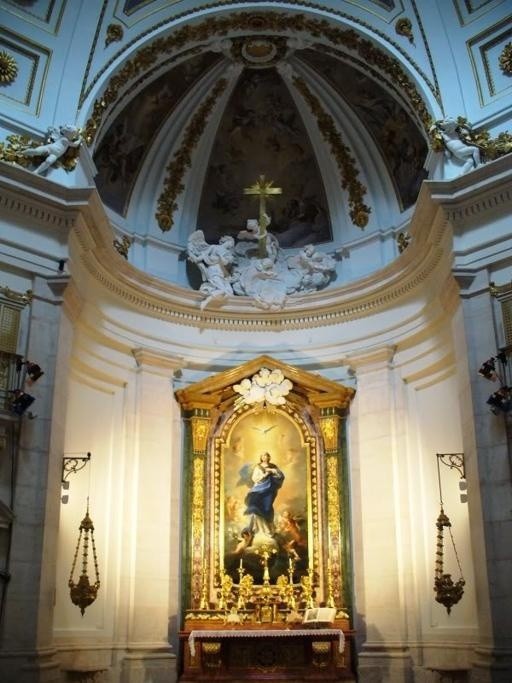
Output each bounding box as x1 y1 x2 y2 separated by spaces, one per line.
289 557 292 569
308 554 314 569
240 558 242 569
221 559 223 570
204 559 206 569
328 559 331 569
264 567 268 576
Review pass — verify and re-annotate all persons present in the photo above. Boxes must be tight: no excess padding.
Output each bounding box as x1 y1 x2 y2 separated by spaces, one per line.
227 451 306 560
430 116 485 173
189 217 338 313
24 124 83 174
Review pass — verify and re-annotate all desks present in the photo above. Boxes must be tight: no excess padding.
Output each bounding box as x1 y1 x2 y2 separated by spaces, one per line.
188 628 346 672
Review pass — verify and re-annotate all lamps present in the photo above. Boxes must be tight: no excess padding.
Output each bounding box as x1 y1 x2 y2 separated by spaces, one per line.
63 453 101 616
433 451 466 615
